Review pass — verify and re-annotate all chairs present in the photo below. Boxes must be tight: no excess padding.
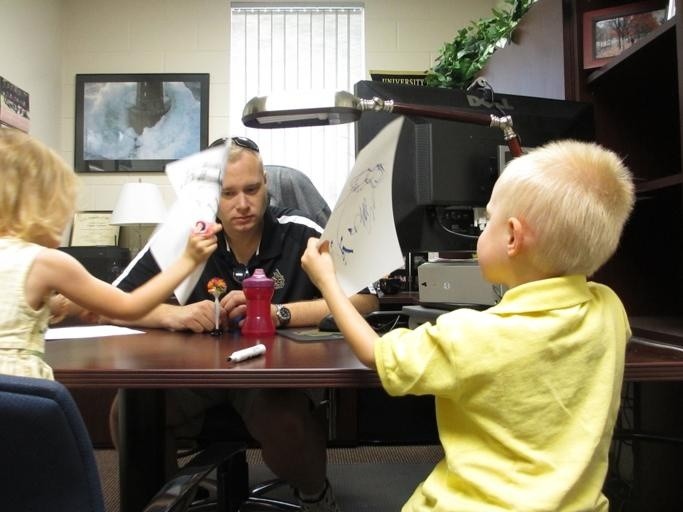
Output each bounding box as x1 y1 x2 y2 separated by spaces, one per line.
0 372 249 512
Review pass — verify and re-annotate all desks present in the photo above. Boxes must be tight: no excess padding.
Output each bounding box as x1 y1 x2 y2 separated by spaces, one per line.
35 317 683 512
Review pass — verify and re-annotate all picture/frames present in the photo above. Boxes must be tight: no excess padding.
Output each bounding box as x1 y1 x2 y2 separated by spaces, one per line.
68 70 211 174
578 1 672 72
66 207 123 247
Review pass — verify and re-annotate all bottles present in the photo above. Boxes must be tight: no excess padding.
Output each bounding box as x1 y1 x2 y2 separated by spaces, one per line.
238 268 276 337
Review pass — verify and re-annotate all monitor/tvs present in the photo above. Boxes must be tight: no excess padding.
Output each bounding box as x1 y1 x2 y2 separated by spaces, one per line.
354 78 594 262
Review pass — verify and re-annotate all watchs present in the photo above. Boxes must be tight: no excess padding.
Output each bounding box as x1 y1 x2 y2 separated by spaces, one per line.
273 303 291 329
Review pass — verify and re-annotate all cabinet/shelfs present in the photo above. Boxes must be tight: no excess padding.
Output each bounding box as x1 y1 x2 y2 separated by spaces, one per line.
558 0 683 354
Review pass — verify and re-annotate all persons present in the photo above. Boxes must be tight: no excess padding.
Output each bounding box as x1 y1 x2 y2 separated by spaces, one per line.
299 134 638 511
0 122 223 382
105 135 382 511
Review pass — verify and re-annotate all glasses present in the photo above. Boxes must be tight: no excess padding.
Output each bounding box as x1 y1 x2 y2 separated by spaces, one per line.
208 136 258 152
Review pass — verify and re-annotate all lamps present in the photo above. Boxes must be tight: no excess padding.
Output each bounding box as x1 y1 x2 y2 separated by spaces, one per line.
235 84 529 169
107 177 168 265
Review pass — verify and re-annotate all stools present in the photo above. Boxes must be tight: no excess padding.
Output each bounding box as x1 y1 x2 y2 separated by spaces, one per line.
168 396 332 511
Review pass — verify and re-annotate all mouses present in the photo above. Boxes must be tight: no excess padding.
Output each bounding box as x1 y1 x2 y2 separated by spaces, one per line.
318 315 340 332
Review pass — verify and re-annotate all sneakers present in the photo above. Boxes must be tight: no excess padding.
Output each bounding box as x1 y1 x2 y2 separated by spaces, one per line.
294 476 340 512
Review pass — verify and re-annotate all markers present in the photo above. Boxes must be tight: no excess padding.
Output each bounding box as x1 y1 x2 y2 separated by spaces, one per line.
227 344 267 362
